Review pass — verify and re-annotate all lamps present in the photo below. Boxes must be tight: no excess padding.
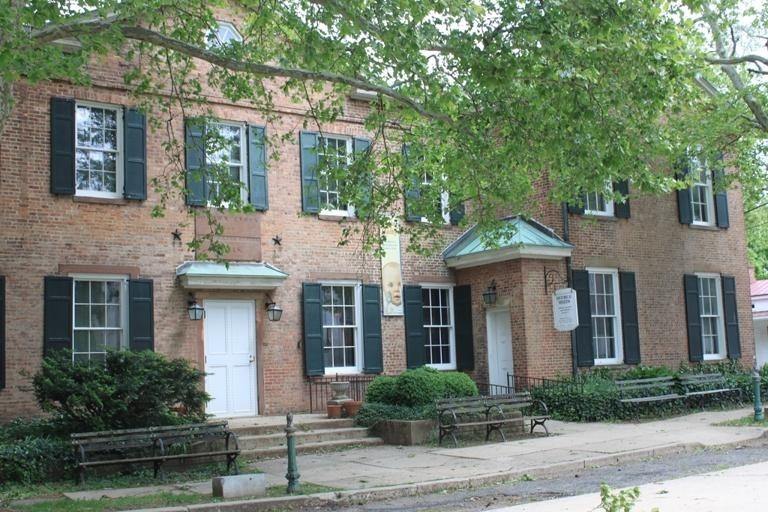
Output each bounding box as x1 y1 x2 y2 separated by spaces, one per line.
188 292 205 322
482 284 497 307
265 292 282 321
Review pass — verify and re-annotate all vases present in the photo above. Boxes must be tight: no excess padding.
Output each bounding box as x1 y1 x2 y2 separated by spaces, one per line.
327 380 354 405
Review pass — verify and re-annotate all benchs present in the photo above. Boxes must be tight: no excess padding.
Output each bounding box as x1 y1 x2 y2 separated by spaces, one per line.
437 390 551 445
68 420 240 483
613 371 742 417
